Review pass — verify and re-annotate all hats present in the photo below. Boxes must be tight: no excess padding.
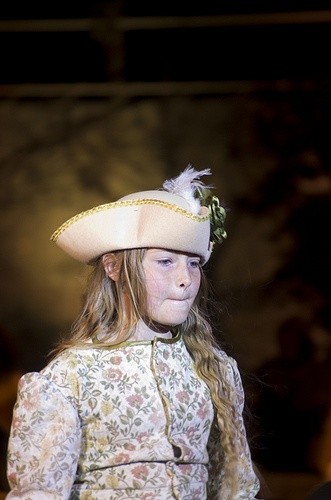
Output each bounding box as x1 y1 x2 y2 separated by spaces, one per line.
49 164 229 266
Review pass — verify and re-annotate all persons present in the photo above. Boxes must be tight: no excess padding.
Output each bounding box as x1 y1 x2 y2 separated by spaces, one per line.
6 190 261 500
